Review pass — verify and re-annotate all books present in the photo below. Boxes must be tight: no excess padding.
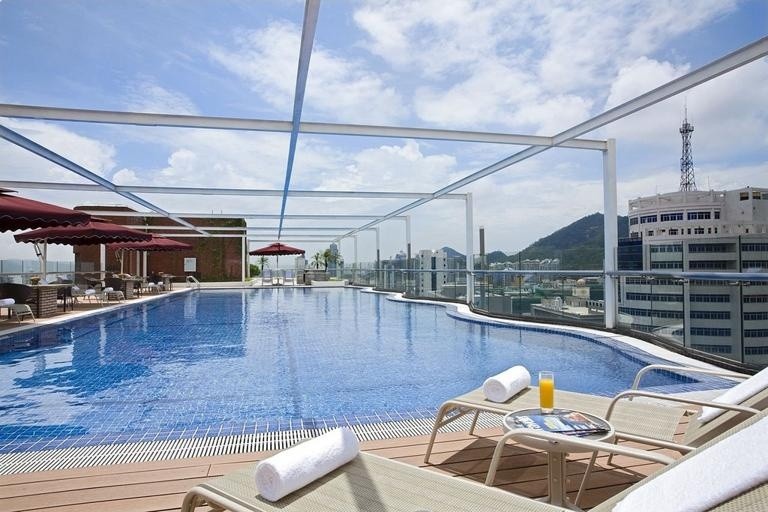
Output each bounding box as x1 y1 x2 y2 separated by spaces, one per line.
514 411 610 438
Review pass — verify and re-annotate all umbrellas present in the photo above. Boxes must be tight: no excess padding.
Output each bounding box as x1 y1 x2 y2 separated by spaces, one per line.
249 242 305 284
0 186 91 233
105 230 193 283
13 216 153 281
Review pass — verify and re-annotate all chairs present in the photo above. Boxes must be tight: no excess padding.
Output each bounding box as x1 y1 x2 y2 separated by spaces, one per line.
423 365 767 506
283 269 295 286
261 269 273 285
140 282 162 294
70 284 126 307
180 406 768 510
0 304 35 324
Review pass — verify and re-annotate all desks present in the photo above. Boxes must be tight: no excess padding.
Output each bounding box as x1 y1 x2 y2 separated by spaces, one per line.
122 279 144 298
161 276 184 291
503 407 615 512
18 283 75 318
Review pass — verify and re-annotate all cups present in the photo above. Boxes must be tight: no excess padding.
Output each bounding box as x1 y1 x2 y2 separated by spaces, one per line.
538 369 555 415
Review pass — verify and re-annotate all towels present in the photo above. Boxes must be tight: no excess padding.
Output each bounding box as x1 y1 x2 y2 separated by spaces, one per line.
0 298 16 305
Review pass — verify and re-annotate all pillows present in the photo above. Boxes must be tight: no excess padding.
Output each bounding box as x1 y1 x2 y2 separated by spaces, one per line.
483 365 533 403
255 427 358 503
